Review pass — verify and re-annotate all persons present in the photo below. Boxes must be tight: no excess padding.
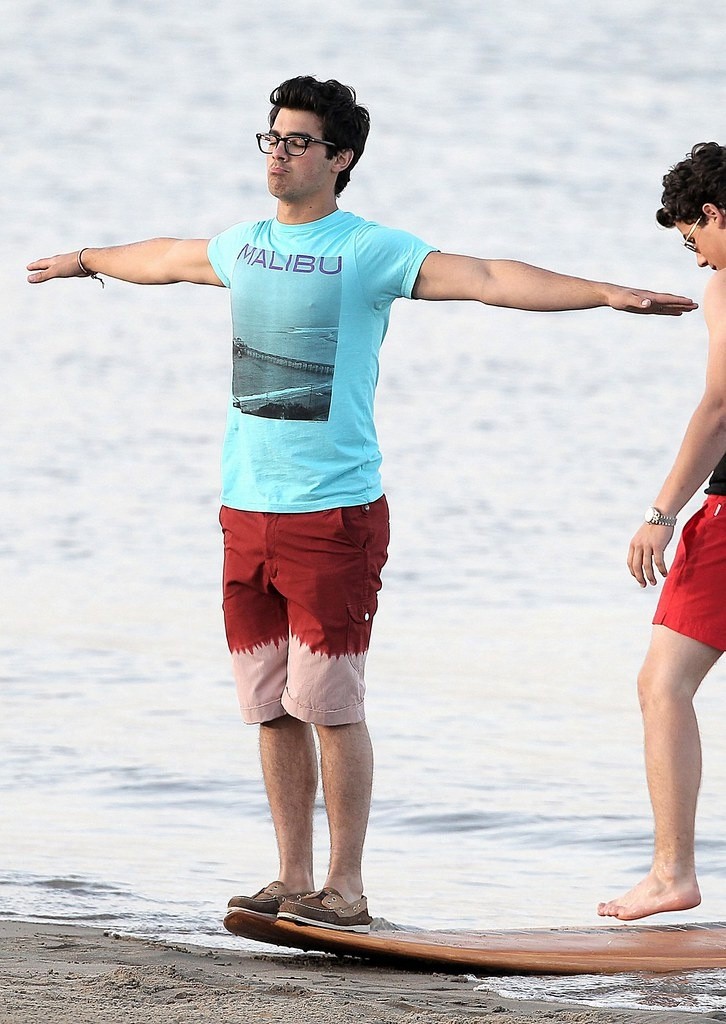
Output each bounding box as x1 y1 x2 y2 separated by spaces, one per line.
26 76 699 932
599 142 726 921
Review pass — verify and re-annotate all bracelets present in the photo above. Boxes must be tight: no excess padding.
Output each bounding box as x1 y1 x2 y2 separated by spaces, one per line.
77 248 104 289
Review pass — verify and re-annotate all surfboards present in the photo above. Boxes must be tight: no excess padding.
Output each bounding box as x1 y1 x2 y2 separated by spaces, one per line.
222 911 726 972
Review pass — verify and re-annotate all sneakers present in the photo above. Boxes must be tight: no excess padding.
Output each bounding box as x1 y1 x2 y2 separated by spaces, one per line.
276 887 373 933
227 881 316 913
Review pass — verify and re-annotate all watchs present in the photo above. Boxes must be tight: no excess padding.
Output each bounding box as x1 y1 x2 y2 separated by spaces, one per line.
644 506 677 526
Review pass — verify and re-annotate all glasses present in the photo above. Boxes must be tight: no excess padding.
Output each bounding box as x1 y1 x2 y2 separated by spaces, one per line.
683 215 703 252
256 133 337 156
